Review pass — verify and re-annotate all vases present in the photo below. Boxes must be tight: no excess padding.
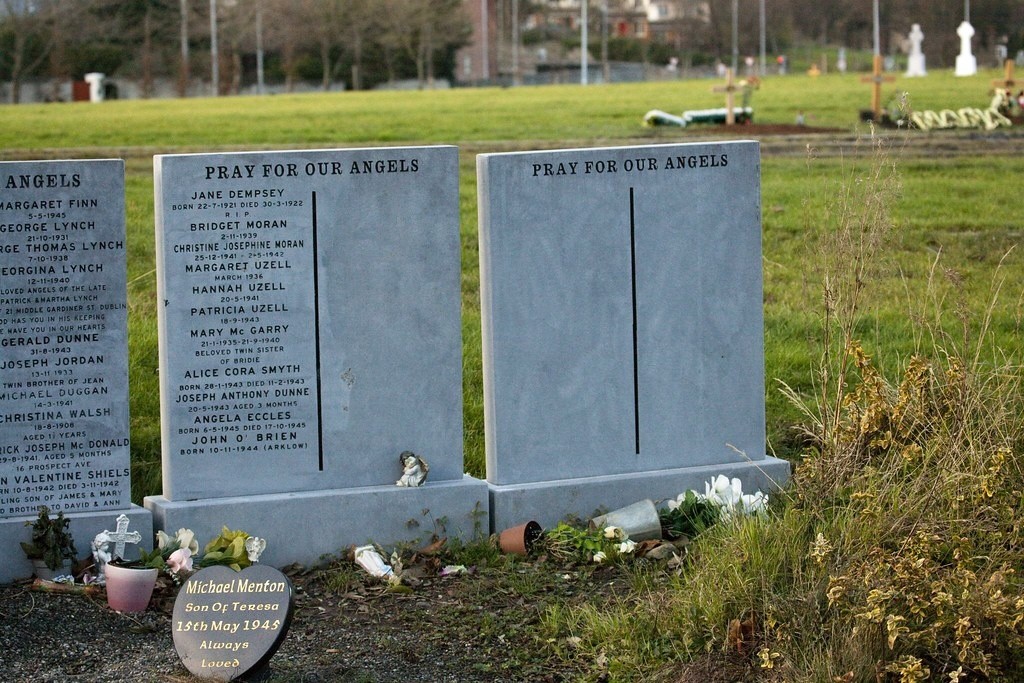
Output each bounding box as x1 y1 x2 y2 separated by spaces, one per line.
588 498 662 543
499 520 544 553
105 558 158 610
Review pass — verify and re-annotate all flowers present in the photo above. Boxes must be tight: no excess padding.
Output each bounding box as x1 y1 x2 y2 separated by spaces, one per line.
531 512 637 564
653 473 772 536
118 525 266 585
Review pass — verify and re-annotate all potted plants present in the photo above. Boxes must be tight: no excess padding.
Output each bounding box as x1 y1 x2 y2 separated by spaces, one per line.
19 506 78 579
